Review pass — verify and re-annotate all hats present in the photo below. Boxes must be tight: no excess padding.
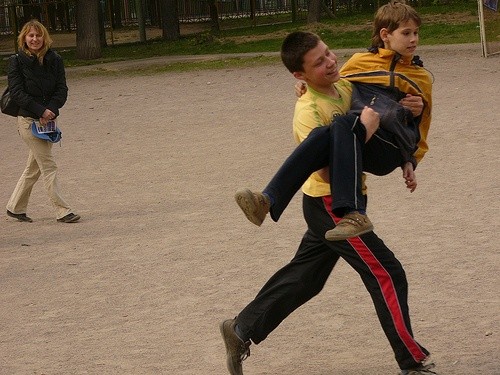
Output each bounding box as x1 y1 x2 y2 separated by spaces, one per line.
32 121 62 143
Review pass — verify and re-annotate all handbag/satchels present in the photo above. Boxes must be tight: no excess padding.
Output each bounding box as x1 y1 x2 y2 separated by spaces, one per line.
0 54 22 117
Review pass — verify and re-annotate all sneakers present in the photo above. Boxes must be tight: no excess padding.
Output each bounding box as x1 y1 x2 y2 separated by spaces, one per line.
219 316 252 375
325 213 374 240
235 187 270 226
399 368 438 375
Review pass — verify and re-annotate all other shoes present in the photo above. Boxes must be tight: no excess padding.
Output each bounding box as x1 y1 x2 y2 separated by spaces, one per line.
57 213 81 223
7 209 32 222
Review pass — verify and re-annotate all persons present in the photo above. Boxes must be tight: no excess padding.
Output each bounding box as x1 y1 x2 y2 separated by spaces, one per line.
218 30 439 375
6 20 81 223
236 3 432 240
